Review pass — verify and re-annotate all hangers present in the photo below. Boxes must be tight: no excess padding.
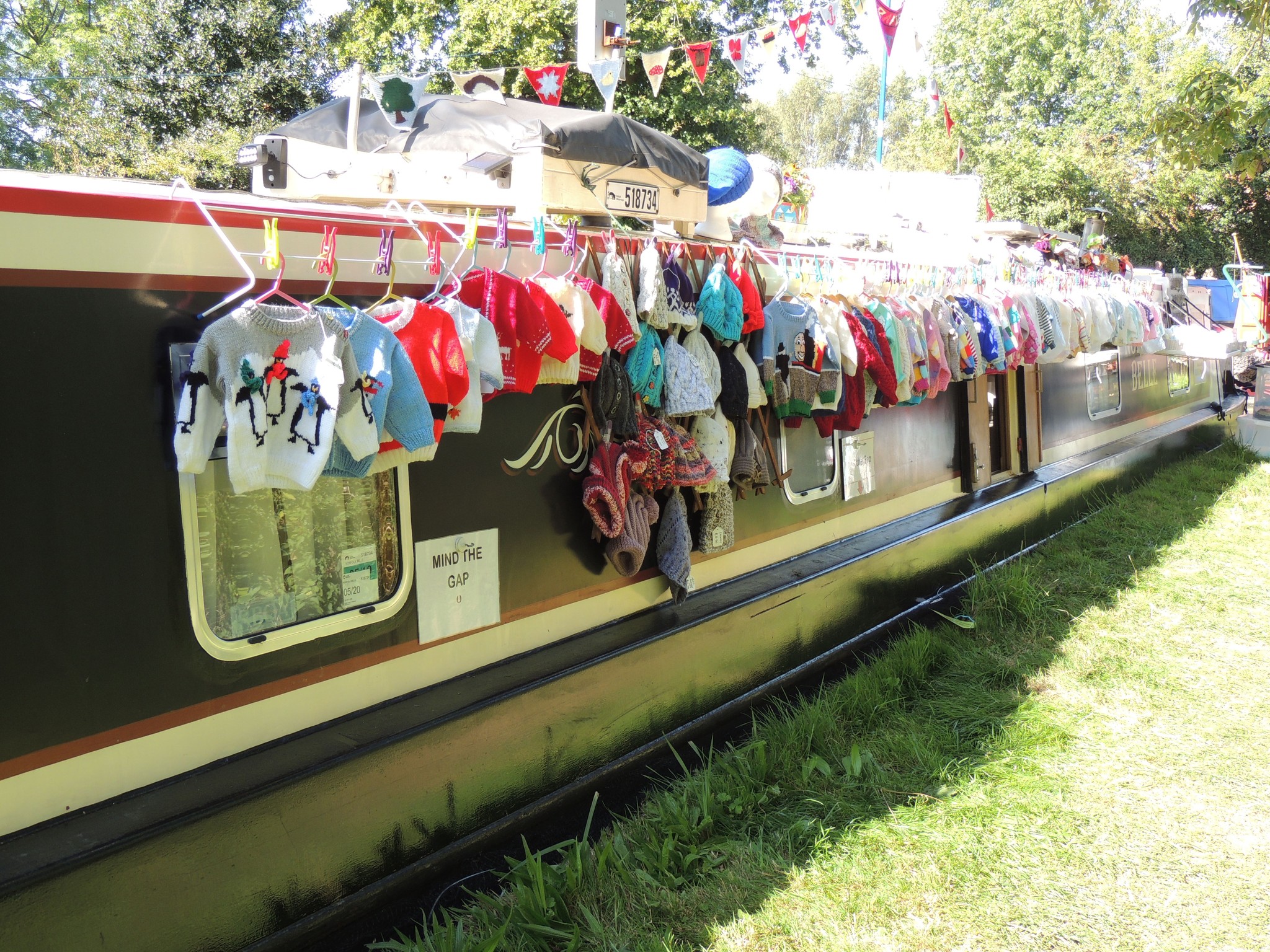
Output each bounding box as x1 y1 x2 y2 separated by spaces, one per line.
253 239 1153 315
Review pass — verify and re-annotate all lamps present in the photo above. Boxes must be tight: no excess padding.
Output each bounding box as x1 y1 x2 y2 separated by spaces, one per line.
459 152 513 189
238 139 287 189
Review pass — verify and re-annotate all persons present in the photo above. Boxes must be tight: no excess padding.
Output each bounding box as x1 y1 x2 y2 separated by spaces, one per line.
1200 268 1217 280
1154 260 1163 272
1184 267 1196 280
1088 239 1103 252
694 148 782 242
